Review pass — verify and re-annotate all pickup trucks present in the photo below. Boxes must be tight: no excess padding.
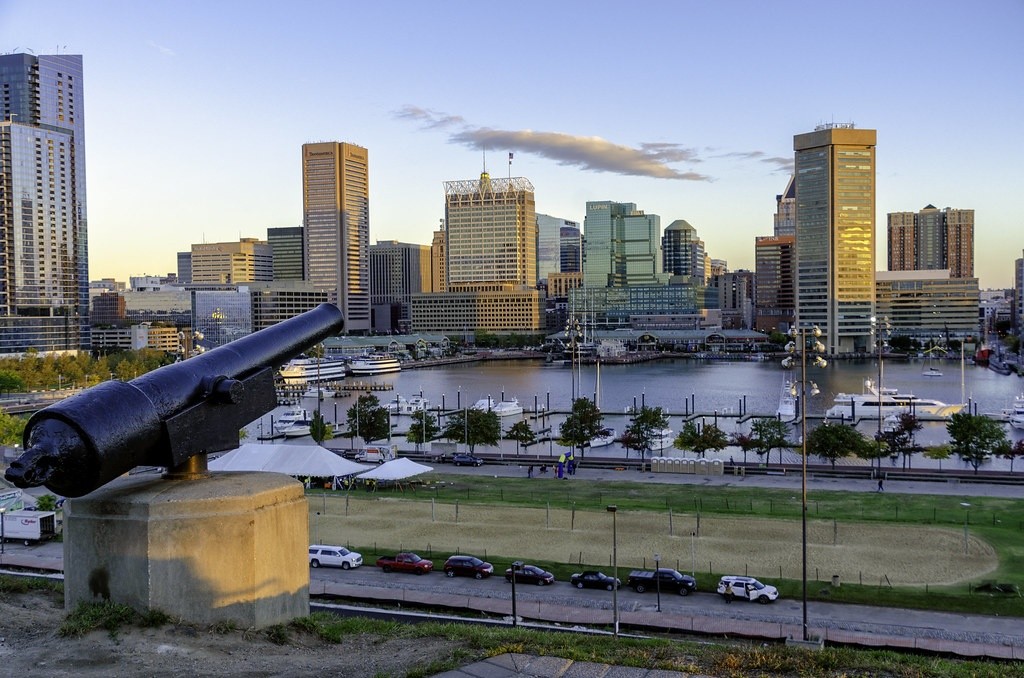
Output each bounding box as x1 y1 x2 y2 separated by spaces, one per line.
628 566 698 596
375 552 434 576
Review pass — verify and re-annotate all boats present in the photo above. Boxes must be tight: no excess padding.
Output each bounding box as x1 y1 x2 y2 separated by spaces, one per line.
825 340 969 420
488 396 524 417
273 405 312 437
971 341 1012 378
278 356 346 385
469 394 495 413
921 366 944 375
301 388 335 398
1001 391 1024 420
643 426 677 450
345 354 402 375
588 427 617 448
382 396 433 414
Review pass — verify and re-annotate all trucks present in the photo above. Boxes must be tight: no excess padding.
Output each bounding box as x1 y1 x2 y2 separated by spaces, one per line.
354 443 397 465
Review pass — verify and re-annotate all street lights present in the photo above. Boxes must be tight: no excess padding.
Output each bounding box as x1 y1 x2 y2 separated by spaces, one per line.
606 503 620 643
0 507 6 554
313 343 325 447
869 313 891 481
565 310 584 414
781 322 828 652
510 559 525 628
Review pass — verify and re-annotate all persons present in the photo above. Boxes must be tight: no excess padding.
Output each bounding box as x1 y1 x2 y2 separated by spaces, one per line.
441 453 446 463
878 478 884 491
345 479 348 491
365 479 376 493
730 456 735 466
553 464 558 479
351 476 357 491
528 464 533 478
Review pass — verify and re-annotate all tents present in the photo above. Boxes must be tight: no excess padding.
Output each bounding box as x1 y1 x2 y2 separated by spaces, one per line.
208 443 377 490
353 457 434 481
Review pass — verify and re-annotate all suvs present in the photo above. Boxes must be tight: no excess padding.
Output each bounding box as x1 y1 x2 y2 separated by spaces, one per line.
453 454 484 468
718 576 780 604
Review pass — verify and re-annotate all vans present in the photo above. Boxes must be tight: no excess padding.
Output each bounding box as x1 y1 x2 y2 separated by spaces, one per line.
309 544 363 570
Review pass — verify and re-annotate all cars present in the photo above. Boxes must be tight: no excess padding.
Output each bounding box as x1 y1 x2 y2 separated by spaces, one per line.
22 497 68 511
569 569 623 590
503 565 556 585
444 556 495 580
